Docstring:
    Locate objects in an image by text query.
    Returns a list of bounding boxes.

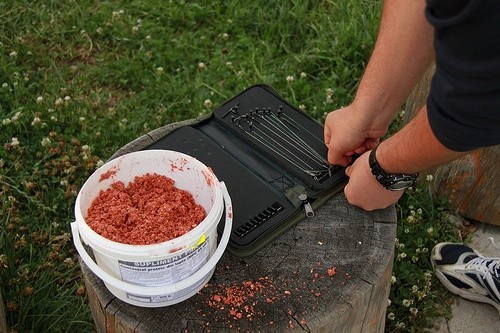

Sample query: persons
[324,0,500,313]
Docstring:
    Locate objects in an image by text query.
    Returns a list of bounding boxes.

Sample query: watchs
[369,144,419,191]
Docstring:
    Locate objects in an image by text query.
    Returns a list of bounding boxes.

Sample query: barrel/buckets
[69,150,233,308]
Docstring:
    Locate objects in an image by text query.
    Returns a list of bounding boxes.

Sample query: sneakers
[431,242,500,315]
